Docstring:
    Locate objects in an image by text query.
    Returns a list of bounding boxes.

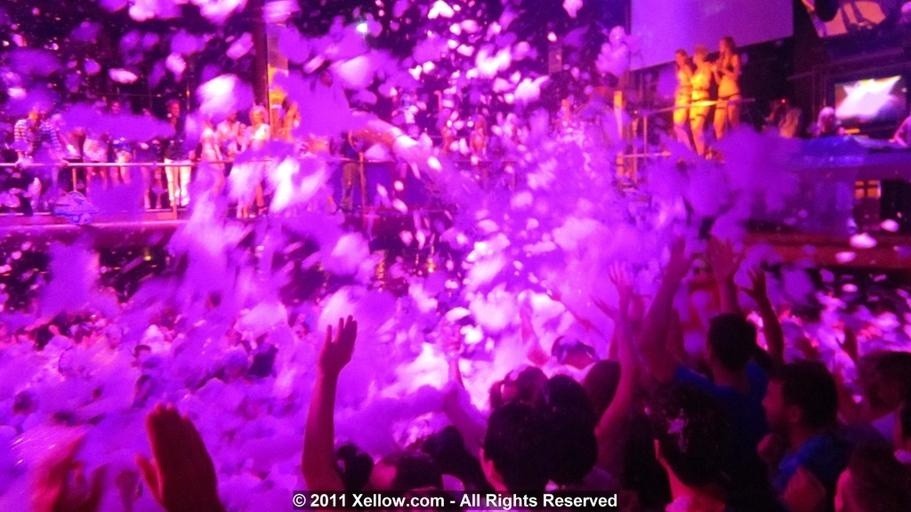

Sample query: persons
[0,35,909,512]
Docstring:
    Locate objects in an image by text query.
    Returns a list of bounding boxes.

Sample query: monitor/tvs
[833,73,901,125]
